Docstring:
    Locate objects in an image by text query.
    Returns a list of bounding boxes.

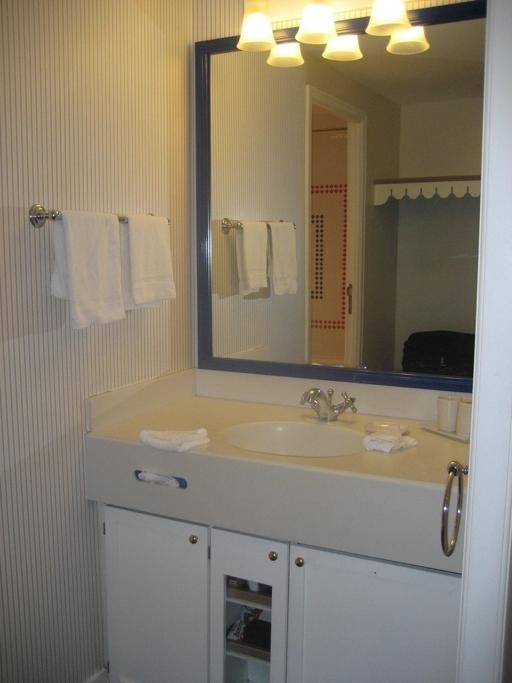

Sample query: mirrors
[194,15,486,392]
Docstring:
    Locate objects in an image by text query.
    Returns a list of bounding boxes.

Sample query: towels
[237,220,269,299]
[362,432,419,455]
[138,427,211,454]
[124,213,179,309]
[266,220,300,297]
[50,208,127,328]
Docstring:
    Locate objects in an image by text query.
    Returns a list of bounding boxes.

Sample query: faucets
[299,387,358,423]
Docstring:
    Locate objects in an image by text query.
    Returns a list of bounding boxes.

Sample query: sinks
[222,420,369,457]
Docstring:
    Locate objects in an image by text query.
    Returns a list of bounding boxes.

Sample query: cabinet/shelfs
[100,502,210,683]
[210,525,462,682]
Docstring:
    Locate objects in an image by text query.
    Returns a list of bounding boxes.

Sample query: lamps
[235,0,412,51]
[266,25,430,68]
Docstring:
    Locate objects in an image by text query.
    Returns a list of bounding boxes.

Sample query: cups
[435,395,460,432]
[456,400,472,438]
